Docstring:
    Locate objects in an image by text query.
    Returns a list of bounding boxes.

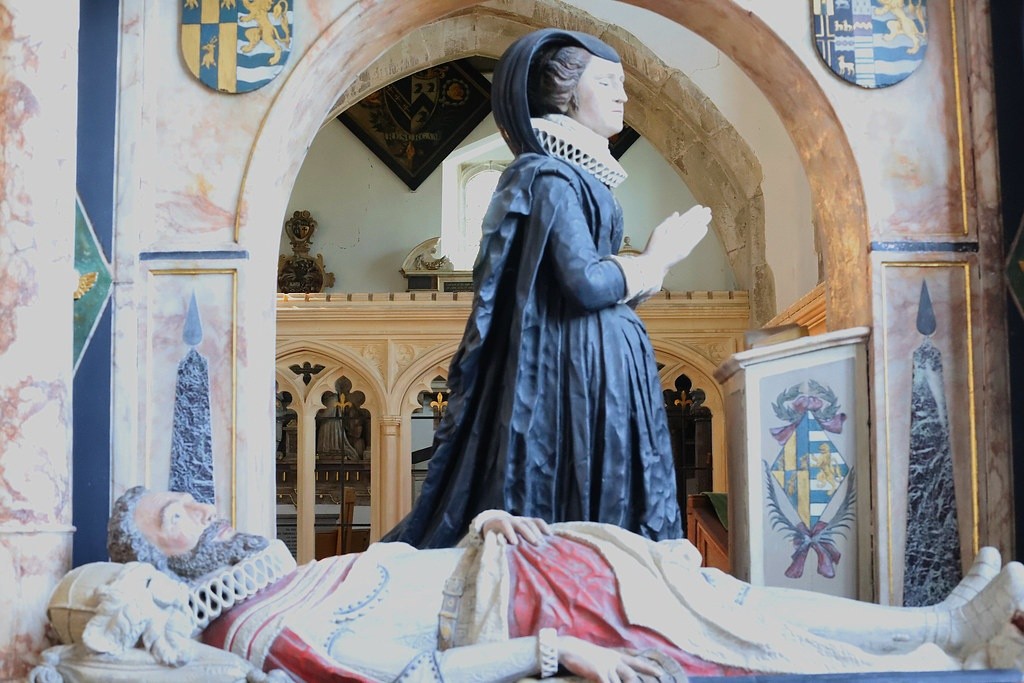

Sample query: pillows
[43,559,162,647]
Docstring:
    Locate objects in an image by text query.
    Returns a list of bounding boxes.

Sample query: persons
[106,485,1024,683]
[375,28,713,550]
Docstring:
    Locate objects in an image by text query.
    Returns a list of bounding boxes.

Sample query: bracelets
[538,629,559,681]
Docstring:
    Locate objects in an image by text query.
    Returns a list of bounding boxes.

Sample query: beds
[42,628,1024,683]
